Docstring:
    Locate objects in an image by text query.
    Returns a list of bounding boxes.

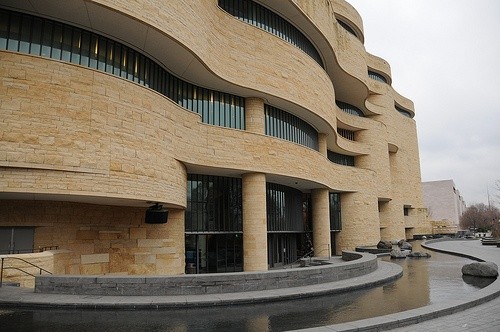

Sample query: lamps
[145,203,169,224]
